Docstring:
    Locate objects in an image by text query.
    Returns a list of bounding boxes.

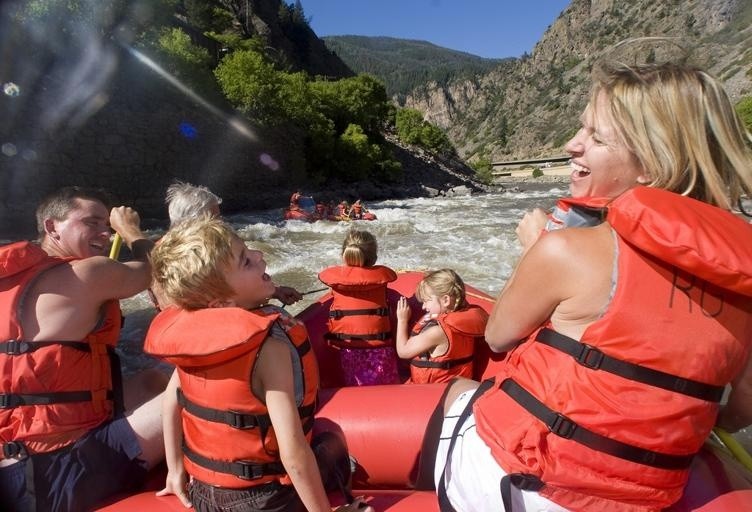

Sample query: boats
[89,272,750,512]
[284,202,379,222]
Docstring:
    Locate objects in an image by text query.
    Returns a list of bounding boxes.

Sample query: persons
[289,191,369,219]
[0,185,175,511]
[318,229,401,386]
[413,35,752,512]
[146,183,302,315]
[144,215,374,512]
[396,269,491,385]
